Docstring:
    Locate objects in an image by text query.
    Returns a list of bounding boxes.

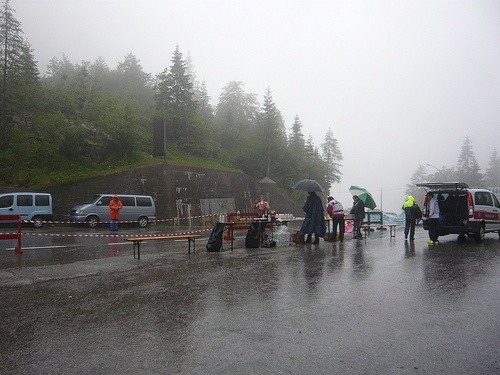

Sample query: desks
[216,219,275,252]
[282,218,360,246]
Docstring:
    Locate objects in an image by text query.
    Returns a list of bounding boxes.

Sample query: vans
[0,192,53,229]
[69,194,157,228]
[416,181,500,243]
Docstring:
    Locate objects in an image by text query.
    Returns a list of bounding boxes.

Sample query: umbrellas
[291,176,323,198]
[348,185,377,211]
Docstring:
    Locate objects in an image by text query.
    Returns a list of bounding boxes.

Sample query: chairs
[436,196,444,201]
[481,195,488,203]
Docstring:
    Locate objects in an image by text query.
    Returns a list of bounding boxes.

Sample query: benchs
[124,234,202,259]
[358,224,399,239]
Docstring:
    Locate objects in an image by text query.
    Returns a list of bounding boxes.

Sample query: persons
[299,192,327,245]
[349,194,365,238]
[109,193,126,231]
[326,196,346,243]
[425,191,441,246]
[402,195,423,241]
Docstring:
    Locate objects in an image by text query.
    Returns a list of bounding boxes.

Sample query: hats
[115,194,119,200]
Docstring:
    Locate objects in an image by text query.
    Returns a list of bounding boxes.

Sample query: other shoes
[111,228,118,231]
[331,236,336,241]
[410,236,415,241]
[339,236,344,242]
[404,232,408,239]
[304,240,311,243]
[352,234,362,239]
[426,238,440,245]
[312,240,319,244]
